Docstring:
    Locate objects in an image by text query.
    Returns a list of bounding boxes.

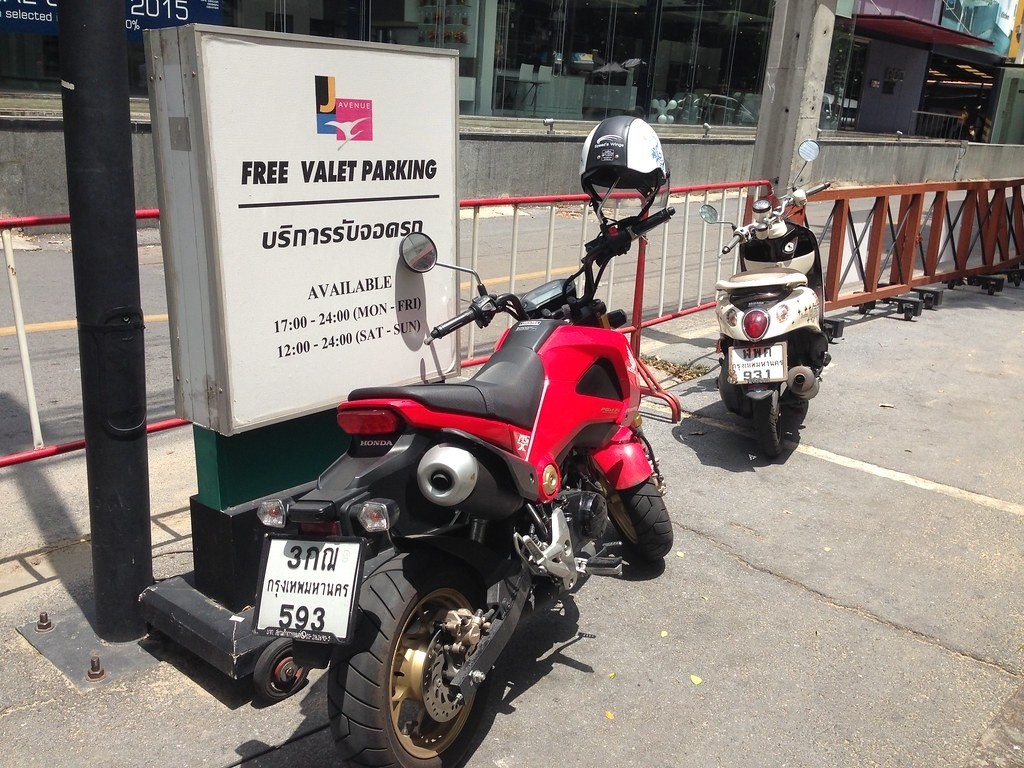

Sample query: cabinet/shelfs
[493,69,586,118]
[585,84,638,114]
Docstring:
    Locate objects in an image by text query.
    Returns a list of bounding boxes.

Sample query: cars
[689,93,754,126]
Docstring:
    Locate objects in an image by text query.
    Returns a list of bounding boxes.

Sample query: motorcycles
[246,176,675,768]
[698,139,831,460]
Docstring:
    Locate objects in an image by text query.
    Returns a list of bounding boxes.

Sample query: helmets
[579,115,667,189]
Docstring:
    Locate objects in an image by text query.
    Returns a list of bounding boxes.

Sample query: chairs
[506,63,553,118]
